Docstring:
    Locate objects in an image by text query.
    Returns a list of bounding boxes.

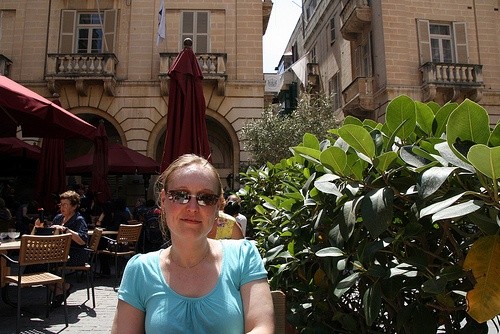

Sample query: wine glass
[0,232,8,241]
[8,232,20,241]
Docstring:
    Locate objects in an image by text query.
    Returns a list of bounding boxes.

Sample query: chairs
[0,216,142,334]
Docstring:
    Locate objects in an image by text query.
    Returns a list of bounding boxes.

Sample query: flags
[155,0,167,47]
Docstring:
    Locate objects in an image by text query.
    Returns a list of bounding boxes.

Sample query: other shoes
[51,281,73,308]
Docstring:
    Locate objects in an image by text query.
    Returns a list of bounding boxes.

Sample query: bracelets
[63,227,69,234]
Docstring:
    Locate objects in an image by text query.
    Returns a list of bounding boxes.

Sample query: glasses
[166,189,219,206]
[59,203,70,208]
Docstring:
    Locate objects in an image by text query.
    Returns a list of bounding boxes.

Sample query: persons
[110,153,277,334]
[0,183,247,307]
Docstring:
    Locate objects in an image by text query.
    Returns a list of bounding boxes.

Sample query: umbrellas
[0,74,160,212]
[160,37,214,175]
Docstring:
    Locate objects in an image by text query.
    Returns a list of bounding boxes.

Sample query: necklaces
[170,246,210,269]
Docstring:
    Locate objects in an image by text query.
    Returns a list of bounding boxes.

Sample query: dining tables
[87,230,119,281]
[0,239,60,301]
